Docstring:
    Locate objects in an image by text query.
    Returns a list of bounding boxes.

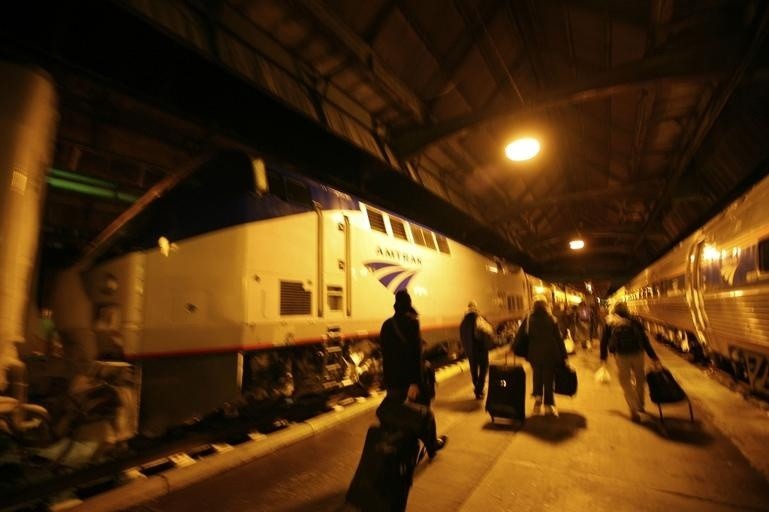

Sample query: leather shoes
[423,436,448,460]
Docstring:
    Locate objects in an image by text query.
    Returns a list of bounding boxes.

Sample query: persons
[459,298,487,401]
[598,300,662,423]
[551,298,611,357]
[380,289,450,462]
[526,300,560,418]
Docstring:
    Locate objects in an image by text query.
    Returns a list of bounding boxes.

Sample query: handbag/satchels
[374,397,434,439]
[513,313,532,357]
[645,361,688,406]
[553,364,578,396]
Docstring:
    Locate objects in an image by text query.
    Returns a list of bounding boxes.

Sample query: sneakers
[534,400,559,418]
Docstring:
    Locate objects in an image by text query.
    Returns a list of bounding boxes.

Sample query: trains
[90,161,590,420]
[601,176,768,396]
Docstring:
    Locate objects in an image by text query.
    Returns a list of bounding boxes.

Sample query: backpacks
[608,312,644,354]
[473,312,497,348]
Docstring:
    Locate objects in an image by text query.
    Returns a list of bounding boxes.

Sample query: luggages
[480,347,527,424]
[345,419,421,512]
